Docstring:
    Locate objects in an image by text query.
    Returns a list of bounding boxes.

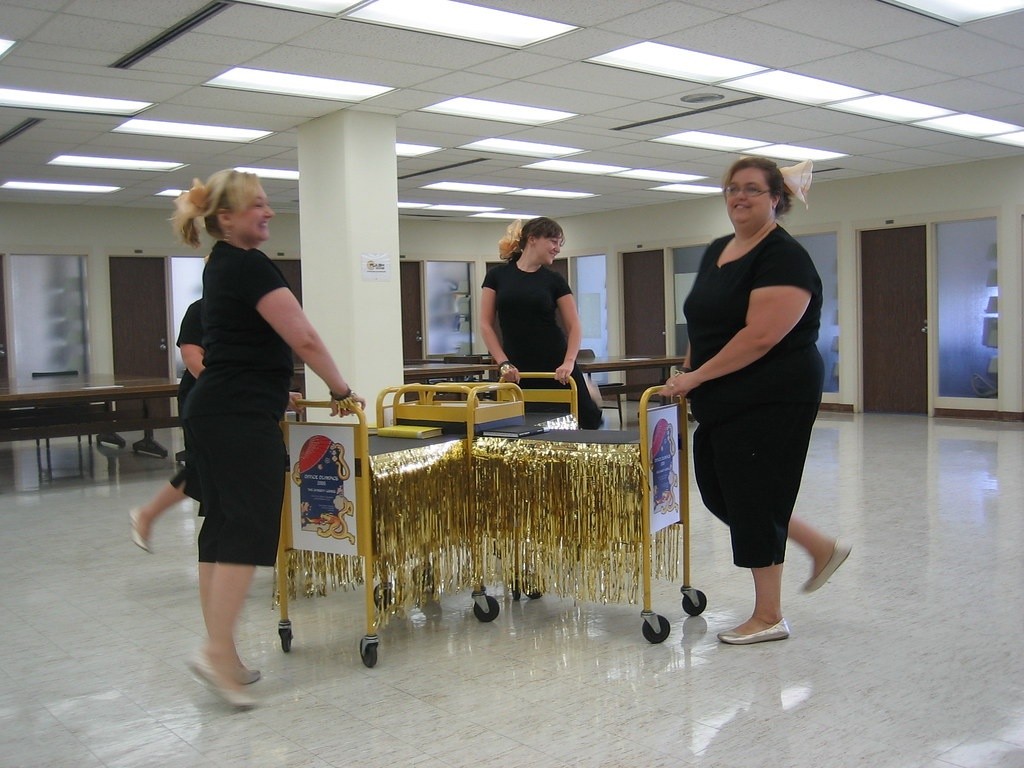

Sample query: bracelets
[329,384,360,410]
[498,360,515,374]
[674,367,691,376]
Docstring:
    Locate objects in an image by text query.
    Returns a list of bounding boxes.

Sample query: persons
[128,298,207,552]
[658,156,853,646]
[171,169,367,708]
[480,216,605,431]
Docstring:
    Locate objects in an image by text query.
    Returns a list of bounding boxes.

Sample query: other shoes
[798,534,853,595]
[183,646,269,707]
[128,506,152,554]
[716,617,790,644]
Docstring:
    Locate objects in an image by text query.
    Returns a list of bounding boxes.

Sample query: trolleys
[364,382,422,429]
[276,384,480,669]
[426,370,580,431]
[466,382,708,646]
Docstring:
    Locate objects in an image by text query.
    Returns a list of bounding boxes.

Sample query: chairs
[444,356,484,397]
[575,348,624,426]
[31,370,92,447]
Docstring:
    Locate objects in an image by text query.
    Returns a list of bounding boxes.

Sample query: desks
[285,345,701,432]
[0,372,186,466]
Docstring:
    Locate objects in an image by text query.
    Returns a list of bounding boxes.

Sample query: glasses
[724,185,771,197]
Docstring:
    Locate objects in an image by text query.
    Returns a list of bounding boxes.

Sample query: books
[377,424,443,439]
[482,425,550,438]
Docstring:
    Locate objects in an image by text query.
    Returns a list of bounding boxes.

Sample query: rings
[670,383,674,388]
